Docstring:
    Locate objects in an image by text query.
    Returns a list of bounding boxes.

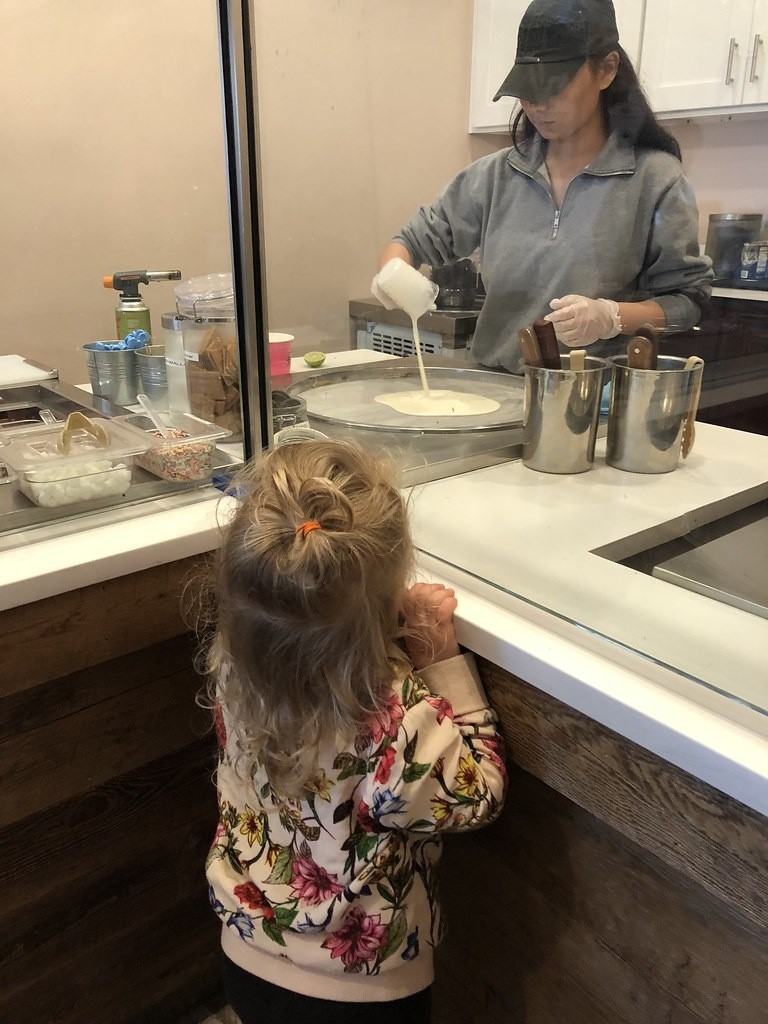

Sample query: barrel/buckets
[134,344,178,411]
[82,339,141,407]
[703,213,764,282]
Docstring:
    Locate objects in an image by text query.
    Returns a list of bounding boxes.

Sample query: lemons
[304,352,326,366]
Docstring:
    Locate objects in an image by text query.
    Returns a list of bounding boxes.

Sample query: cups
[375,256,441,319]
[519,352,612,474]
[606,354,702,474]
[268,332,295,376]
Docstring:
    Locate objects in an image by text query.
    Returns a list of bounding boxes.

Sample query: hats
[492,0,620,103]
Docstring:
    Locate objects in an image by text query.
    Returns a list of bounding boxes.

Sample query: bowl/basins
[436,287,477,311]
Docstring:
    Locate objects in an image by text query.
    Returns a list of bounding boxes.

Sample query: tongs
[680,356,704,459]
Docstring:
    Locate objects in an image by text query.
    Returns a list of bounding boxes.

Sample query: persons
[174,436,507,1023]
[371,0,714,375]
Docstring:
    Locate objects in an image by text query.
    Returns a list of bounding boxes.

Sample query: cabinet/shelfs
[705,298,768,437]
[659,296,726,424]
[468,0,767,136]
